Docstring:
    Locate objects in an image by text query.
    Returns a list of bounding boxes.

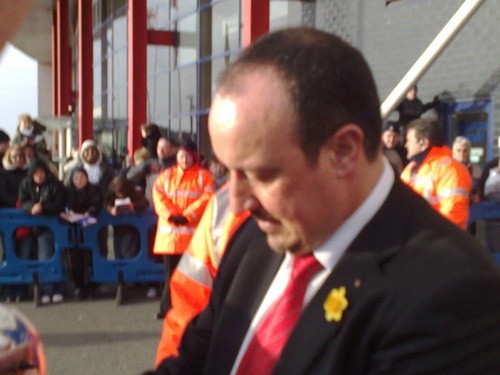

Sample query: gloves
[168,215,187,226]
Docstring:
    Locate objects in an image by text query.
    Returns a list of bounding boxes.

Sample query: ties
[235,254,323,375]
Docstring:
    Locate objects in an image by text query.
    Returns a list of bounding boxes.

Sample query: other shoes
[156,311,166,319]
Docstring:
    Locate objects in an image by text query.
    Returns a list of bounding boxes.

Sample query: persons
[140,24,500,375]
[0,113,229,320]
[382,84,500,232]
